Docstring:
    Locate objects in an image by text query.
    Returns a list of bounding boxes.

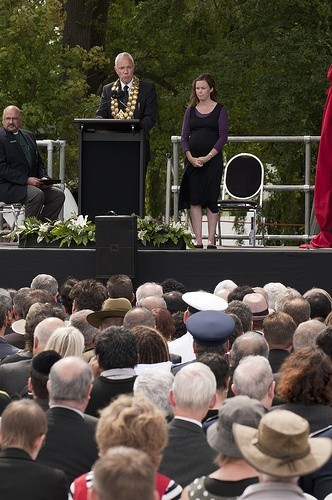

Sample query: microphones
[82,94,116,118]
[116,95,135,120]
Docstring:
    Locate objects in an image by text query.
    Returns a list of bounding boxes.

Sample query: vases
[19,234,185,249]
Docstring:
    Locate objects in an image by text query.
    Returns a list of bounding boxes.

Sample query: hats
[232,409,332,477]
[251,287,269,307]
[207,395,272,457]
[32,349,63,375]
[264,282,286,309]
[213,280,238,303]
[86,298,133,329]
[243,293,275,321]
[185,310,235,346]
[12,302,51,335]
[182,292,229,313]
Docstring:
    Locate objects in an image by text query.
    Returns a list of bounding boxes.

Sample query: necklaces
[110,75,140,120]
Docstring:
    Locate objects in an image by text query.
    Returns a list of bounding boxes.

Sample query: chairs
[217,152,267,248]
[0,203,26,246]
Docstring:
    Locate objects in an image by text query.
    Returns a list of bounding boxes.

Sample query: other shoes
[207,244,217,249]
[194,244,203,248]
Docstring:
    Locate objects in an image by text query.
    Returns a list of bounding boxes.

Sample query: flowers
[3,215,197,250]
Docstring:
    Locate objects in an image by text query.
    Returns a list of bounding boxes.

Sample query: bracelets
[209,151,213,157]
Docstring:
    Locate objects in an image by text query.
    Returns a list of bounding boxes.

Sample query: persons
[95,51,158,178]
[0,273,332,500]
[181,72,228,249]
[0,104,65,226]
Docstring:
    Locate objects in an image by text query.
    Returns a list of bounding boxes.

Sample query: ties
[123,86,129,113]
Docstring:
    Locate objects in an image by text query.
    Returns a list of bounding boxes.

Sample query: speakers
[94,214,137,279]
